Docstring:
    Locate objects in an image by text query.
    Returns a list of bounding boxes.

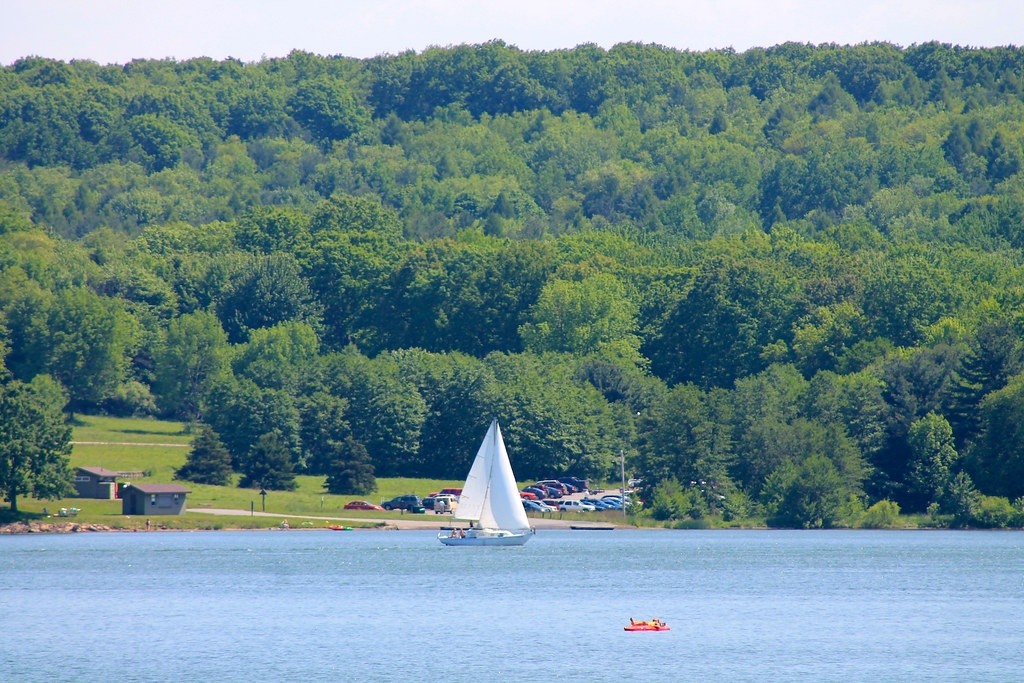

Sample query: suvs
[381,495,422,512]
[423,493,457,510]
[430,488,463,500]
[434,495,459,514]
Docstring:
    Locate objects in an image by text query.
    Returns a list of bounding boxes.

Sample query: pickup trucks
[558,476,589,492]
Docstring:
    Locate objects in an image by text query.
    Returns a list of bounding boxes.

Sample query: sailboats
[437,410,536,547]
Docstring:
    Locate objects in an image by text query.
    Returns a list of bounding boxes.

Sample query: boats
[624,618,670,631]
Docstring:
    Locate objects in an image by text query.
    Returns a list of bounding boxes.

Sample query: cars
[519,490,536,500]
[522,486,547,500]
[563,482,578,495]
[343,500,376,509]
[522,478,647,514]
